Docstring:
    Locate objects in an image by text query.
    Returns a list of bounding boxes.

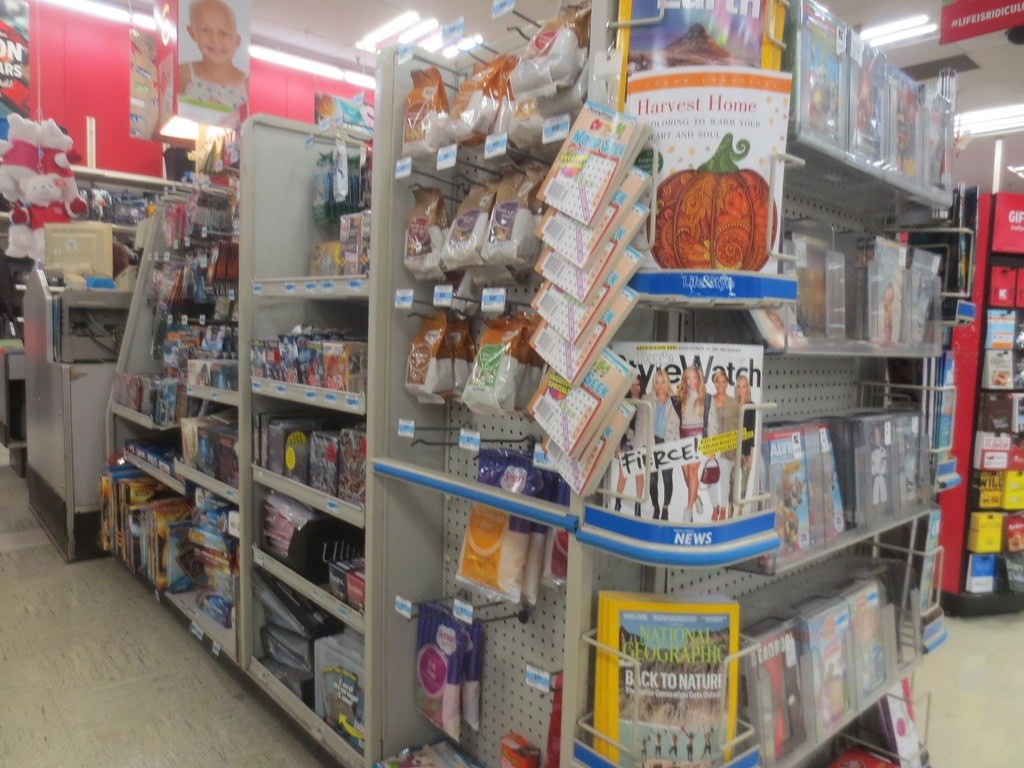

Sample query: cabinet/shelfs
[0,1,1023,768]
